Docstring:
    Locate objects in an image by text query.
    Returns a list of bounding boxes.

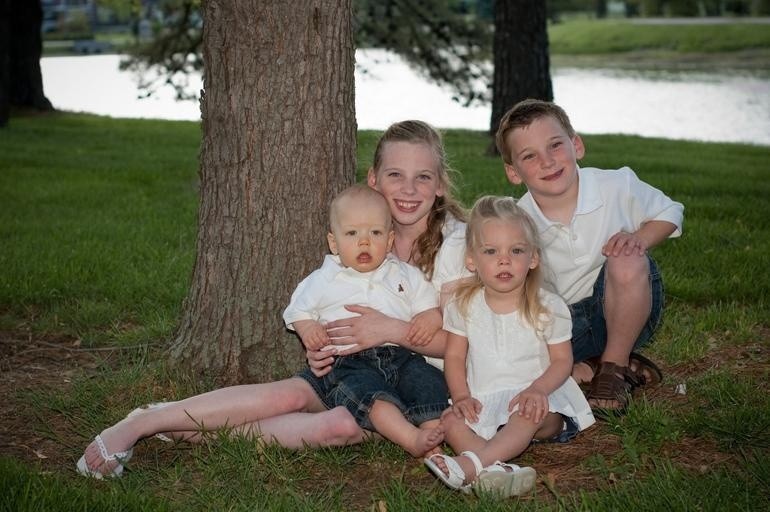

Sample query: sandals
[473,459,536,500]
[424,451,484,493]
[586,352,662,418]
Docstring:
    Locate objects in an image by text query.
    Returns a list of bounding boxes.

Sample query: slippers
[77,402,174,481]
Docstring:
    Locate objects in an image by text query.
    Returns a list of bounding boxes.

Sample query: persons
[494,95,684,419]
[279,180,454,460]
[77,119,484,484]
[424,193,600,506]
